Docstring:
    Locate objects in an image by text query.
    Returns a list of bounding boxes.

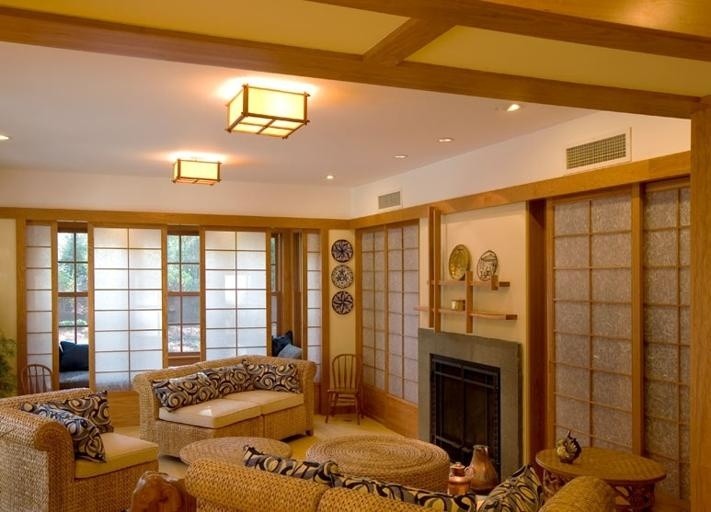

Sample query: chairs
[324,352,364,425]
[21,362,53,393]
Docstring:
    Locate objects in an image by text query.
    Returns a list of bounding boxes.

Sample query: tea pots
[449,462,477,496]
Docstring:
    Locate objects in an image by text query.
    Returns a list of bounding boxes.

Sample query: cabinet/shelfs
[412,278,519,334]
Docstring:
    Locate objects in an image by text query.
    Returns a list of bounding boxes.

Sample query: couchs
[182,456,617,512]
[0,386,160,512]
[131,354,317,463]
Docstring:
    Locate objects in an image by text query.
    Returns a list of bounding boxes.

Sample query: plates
[477,250,498,282]
[448,244,470,280]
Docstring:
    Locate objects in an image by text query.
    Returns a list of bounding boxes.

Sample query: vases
[466,444,499,497]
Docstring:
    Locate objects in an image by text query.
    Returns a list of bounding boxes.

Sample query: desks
[536,446,667,510]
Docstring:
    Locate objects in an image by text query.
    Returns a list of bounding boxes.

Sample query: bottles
[469,444,500,493]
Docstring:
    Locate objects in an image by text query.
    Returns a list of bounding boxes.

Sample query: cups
[452,300,465,311]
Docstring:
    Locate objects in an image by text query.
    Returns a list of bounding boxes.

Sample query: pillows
[22,400,107,467]
[151,371,224,413]
[242,442,340,494]
[330,469,477,511]
[478,463,547,512]
[37,391,115,434]
[59,339,89,371]
[201,362,254,394]
[270,331,303,359]
[242,357,301,396]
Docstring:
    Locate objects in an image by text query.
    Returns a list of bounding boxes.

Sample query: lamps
[223,83,312,139]
[171,157,222,188]
